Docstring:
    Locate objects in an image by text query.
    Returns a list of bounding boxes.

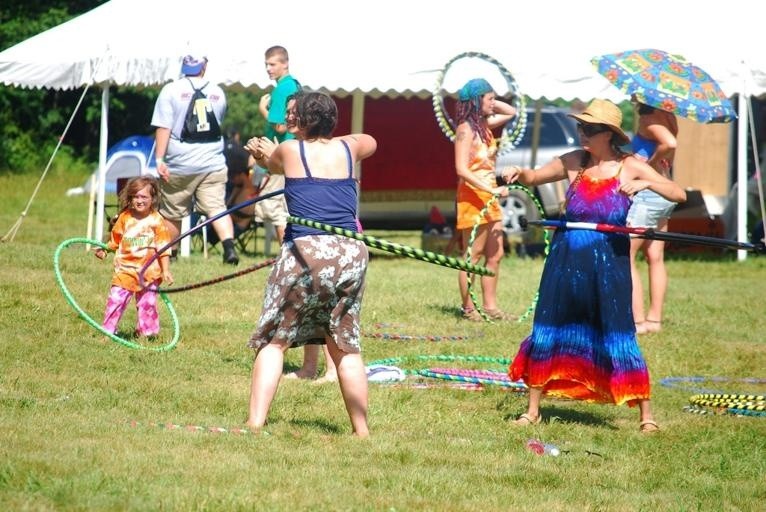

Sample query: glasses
[576,123,606,137]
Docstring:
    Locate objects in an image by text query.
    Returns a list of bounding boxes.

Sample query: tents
[83,134,160,192]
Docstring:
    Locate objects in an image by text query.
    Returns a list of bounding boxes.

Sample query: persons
[150,51,240,267]
[243,91,378,438]
[244,93,341,384]
[625,91,680,336]
[451,77,518,320]
[501,96,688,437]
[253,45,304,245]
[91,175,175,345]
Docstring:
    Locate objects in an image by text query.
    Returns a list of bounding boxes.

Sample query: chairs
[191,184,258,257]
[96,176,129,228]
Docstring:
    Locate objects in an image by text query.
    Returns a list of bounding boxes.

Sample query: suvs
[493,105,587,248]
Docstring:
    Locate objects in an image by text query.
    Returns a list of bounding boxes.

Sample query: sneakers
[223,248,238,264]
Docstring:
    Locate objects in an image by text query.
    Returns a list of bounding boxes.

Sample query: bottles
[527,438,562,456]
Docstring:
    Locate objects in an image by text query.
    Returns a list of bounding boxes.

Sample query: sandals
[512,412,542,426]
[482,305,517,321]
[640,420,658,432]
[461,307,484,322]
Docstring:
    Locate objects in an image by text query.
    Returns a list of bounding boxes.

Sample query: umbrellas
[590,49,739,127]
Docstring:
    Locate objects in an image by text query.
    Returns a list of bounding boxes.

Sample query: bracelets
[254,154,265,161]
[154,157,166,165]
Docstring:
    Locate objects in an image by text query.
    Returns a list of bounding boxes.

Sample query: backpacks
[179,78,221,143]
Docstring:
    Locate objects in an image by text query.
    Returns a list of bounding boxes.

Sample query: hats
[182,55,206,76]
[567,99,632,146]
